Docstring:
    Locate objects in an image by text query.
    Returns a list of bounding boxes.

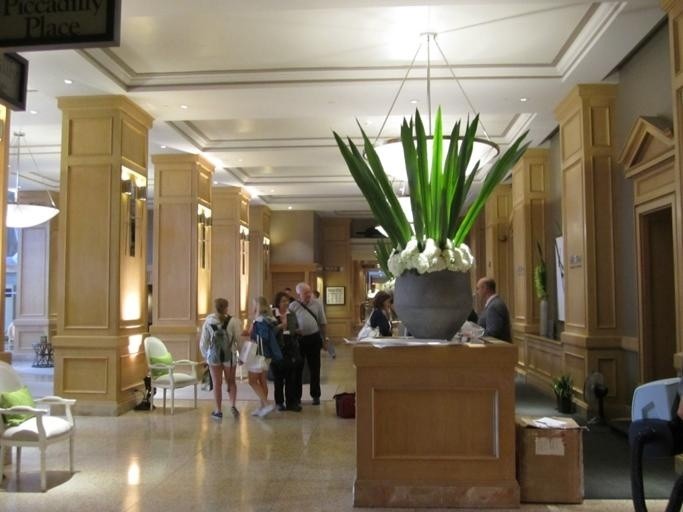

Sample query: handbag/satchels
[271,341,304,375]
[334,392,354,418]
[239,341,257,364]
[248,355,265,373]
[357,323,381,337]
[201,368,213,391]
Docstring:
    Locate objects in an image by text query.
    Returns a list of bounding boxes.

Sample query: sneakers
[212,411,222,418]
[251,408,262,416]
[287,405,301,411]
[277,404,284,411]
[312,398,319,405]
[230,407,239,416]
[260,406,274,417]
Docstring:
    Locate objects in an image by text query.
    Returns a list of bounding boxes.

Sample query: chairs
[0,361,77,491]
[142,334,198,413]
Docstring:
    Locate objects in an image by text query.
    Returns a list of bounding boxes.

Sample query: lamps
[121,177,147,202]
[6,124,57,229]
[365,30,496,179]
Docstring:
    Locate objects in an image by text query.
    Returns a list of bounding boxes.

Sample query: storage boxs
[517,414,587,505]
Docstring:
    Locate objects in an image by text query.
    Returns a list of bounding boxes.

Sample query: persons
[239,295,285,418]
[467,310,478,323]
[199,297,242,420]
[367,291,393,336]
[270,282,335,412]
[476,277,512,342]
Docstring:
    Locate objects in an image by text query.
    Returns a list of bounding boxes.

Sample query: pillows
[150,352,174,376]
[0,386,36,425]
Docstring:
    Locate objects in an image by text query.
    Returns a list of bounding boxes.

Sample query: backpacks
[207,315,234,363]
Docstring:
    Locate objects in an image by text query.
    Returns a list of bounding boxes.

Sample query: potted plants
[330,107,533,340]
[550,373,573,415]
[533,241,549,337]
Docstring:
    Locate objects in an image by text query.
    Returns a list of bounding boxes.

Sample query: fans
[581,371,608,428]
